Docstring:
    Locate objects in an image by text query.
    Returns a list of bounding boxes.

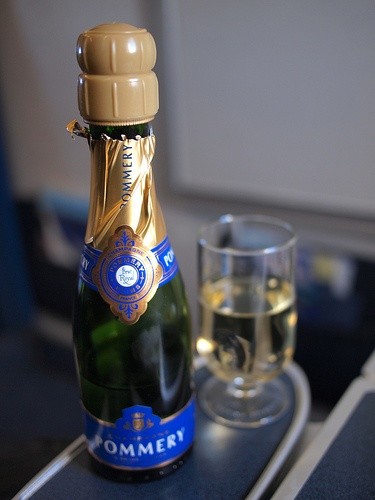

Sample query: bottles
[70,23,196,484]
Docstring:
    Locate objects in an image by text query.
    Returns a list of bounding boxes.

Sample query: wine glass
[195,214,297,429]
